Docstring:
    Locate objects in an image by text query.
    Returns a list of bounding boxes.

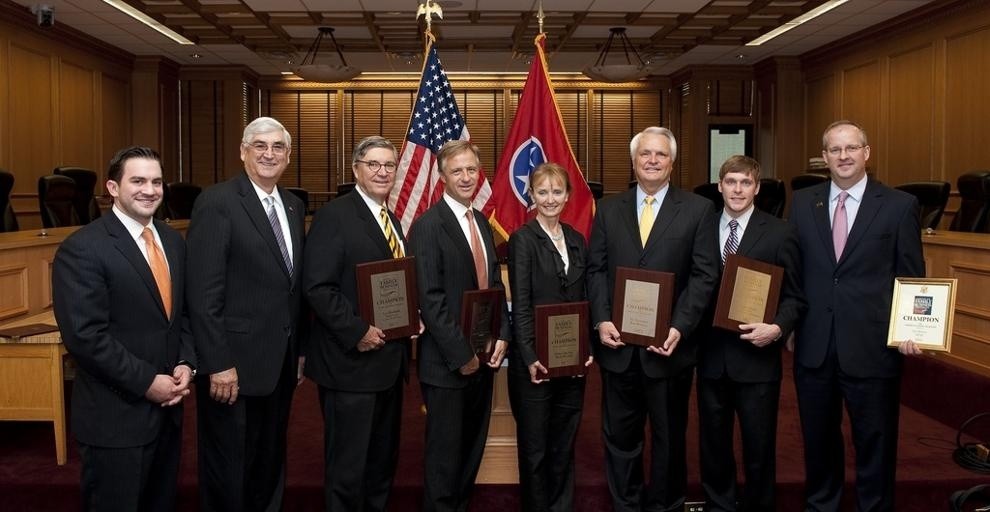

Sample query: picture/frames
[886,277,958,353]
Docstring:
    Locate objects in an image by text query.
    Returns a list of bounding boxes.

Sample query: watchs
[177,359,197,379]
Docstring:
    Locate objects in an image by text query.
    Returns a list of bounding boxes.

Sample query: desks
[0,310,69,466]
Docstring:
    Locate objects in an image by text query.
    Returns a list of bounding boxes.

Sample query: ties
[380,207,404,258]
[832,192,848,261]
[265,196,293,277]
[723,220,739,263]
[142,226,172,321]
[640,196,655,247]
[466,210,487,289]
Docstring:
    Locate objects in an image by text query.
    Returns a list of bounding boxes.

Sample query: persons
[298,135,425,511]
[504,162,594,512]
[585,124,719,511]
[406,141,512,512]
[50,146,198,511]
[693,154,805,512]
[186,115,306,512]
[788,120,937,511]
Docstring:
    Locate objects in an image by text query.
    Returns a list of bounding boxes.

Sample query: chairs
[896,181,951,230]
[588,182,603,200]
[38,174,80,229]
[55,168,102,221]
[285,188,310,208]
[949,172,990,232]
[2,172,21,234]
[166,182,203,220]
[694,183,725,215]
[754,176,786,219]
[335,183,355,197]
[791,174,829,190]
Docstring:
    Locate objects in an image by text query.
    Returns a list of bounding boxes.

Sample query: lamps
[583,27,657,83]
[290,27,361,82]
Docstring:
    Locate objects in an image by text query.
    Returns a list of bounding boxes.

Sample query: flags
[490,31,598,255]
[383,34,494,242]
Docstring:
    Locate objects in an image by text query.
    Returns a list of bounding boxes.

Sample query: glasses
[356,159,398,174]
[249,141,286,155]
[829,144,864,153]
